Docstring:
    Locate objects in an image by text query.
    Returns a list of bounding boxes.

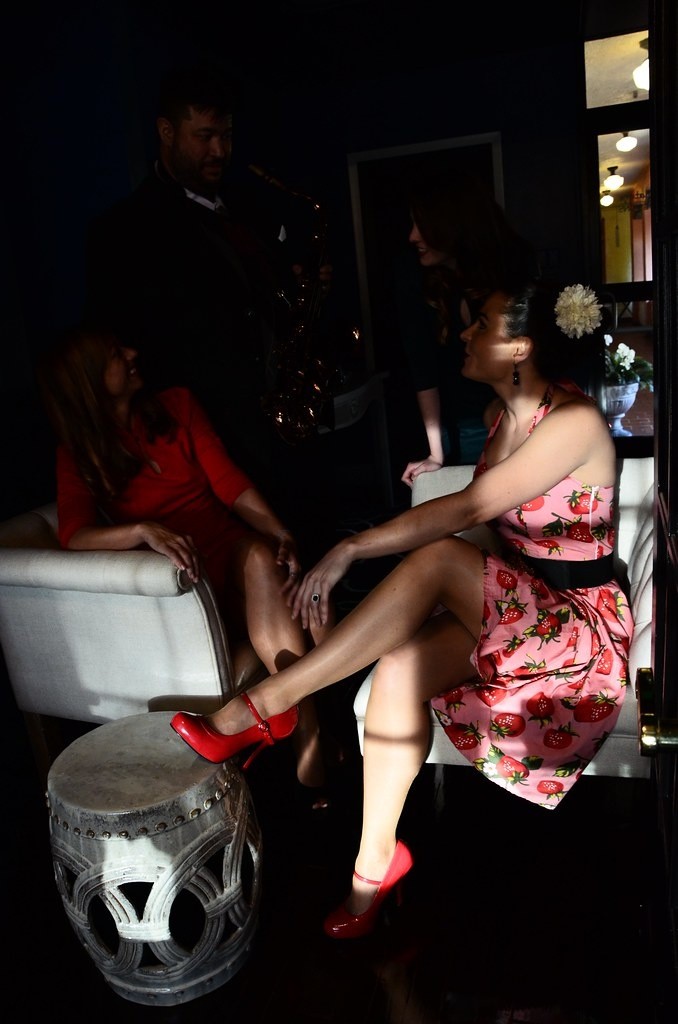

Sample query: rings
[289,573,298,579]
[310,593,319,603]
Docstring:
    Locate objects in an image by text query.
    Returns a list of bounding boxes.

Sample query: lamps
[616,133,638,152]
[604,166,624,190]
[633,38,649,91]
[600,190,614,207]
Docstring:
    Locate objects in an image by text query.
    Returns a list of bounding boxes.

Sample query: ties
[214,206,231,221]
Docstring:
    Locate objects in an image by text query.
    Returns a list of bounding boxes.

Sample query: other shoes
[295,758,333,811]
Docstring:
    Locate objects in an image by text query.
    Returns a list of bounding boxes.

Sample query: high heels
[323,838,416,941]
[169,691,301,770]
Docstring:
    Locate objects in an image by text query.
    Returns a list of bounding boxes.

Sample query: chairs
[353,456,656,819]
[0,501,262,811]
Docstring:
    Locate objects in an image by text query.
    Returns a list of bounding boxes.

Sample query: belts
[517,550,616,593]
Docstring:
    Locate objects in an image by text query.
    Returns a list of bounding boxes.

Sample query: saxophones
[247,161,373,445]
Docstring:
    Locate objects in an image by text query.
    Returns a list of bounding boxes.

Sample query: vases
[585,383,639,437]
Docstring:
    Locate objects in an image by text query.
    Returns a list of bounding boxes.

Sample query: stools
[43,712,271,1008]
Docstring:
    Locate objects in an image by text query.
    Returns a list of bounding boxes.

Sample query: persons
[169,263,635,938]
[35,326,351,822]
[401,185,538,488]
[85,97,265,490]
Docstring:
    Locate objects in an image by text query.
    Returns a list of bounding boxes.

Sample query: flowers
[601,334,653,393]
[554,284,603,339]
